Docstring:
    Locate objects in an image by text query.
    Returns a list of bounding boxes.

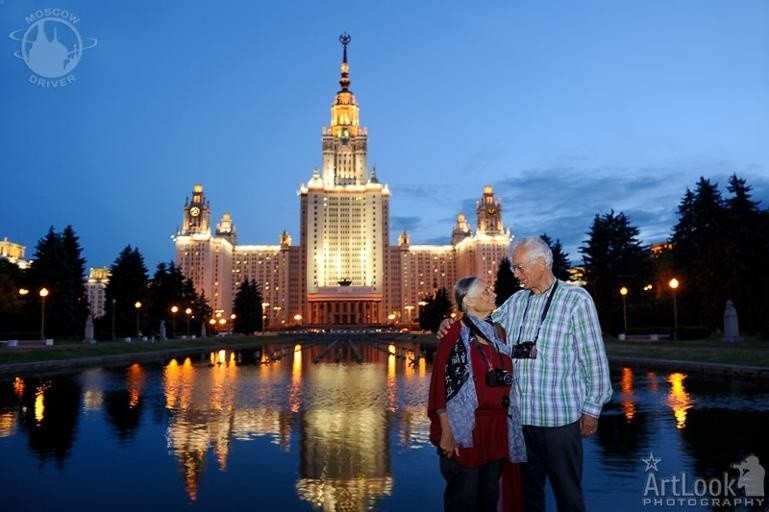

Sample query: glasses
[510,261,531,273]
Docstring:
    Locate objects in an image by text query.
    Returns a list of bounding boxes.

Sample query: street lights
[184,307,192,336]
[38,287,50,345]
[386,300,429,333]
[618,285,628,328]
[170,305,179,339]
[111,298,117,342]
[207,301,302,335]
[668,277,680,340]
[133,301,143,338]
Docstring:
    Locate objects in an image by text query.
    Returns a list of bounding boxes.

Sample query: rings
[590,429,595,434]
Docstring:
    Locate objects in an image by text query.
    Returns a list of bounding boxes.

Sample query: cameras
[511,344,537,359]
[487,368,513,386]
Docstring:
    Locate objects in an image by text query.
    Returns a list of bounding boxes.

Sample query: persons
[423,274,517,512]
[722,300,740,337]
[433,236,614,512]
